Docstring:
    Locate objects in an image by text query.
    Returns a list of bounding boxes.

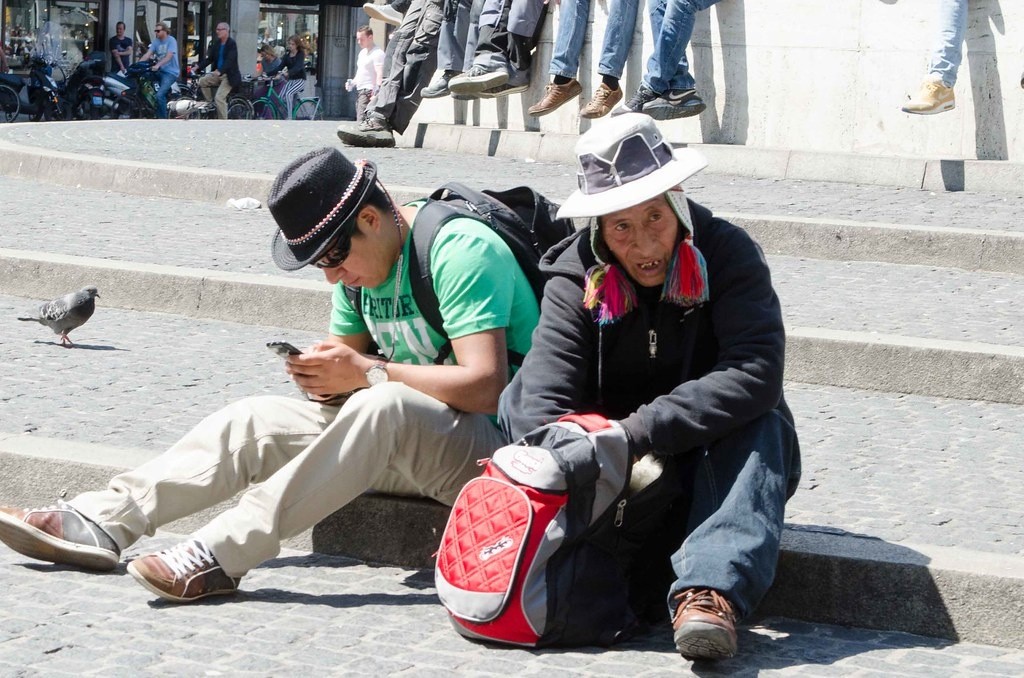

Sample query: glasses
[216,28,226,32]
[309,214,356,270]
[154,29,164,33]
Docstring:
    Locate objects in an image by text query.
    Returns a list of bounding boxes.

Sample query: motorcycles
[0,22,136,122]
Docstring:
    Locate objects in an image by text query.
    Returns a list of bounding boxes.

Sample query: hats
[266,146,377,272]
[554,113,708,219]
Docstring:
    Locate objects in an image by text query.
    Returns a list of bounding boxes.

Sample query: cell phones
[266,342,303,360]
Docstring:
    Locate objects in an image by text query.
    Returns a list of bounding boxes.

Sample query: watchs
[366,360,388,387]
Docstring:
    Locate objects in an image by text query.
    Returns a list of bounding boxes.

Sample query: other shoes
[337,113,396,148]
[528,77,582,117]
[580,82,623,119]
[363,2,404,25]
[199,102,216,111]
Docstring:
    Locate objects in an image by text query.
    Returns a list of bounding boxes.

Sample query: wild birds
[17,285,101,349]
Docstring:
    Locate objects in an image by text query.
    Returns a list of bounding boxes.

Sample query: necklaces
[387,205,405,363]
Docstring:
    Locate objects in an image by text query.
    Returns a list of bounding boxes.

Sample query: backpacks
[430,413,667,648]
[408,182,577,367]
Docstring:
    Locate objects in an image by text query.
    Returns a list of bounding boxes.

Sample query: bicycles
[111,56,254,120]
[0,83,20,123]
[243,70,323,120]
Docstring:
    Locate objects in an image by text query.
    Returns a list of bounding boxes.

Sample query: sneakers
[451,92,478,101]
[609,83,660,119]
[1,489,121,569]
[127,539,240,601]
[899,73,955,114]
[420,70,461,98]
[448,63,508,93]
[669,583,736,661]
[642,88,706,120]
[473,84,531,99]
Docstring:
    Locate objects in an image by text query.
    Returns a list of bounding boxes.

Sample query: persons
[109,21,133,119]
[192,22,241,119]
[345,26,385,124]
[612,0,719,121]
[261,36,307,120]
[498,113,802,659]
[0,40,9,73]
[529,0,638,118]
[880,0,969,116]
[139,23,180,119]
[0,148,539,602]
[337,0,549,146]
